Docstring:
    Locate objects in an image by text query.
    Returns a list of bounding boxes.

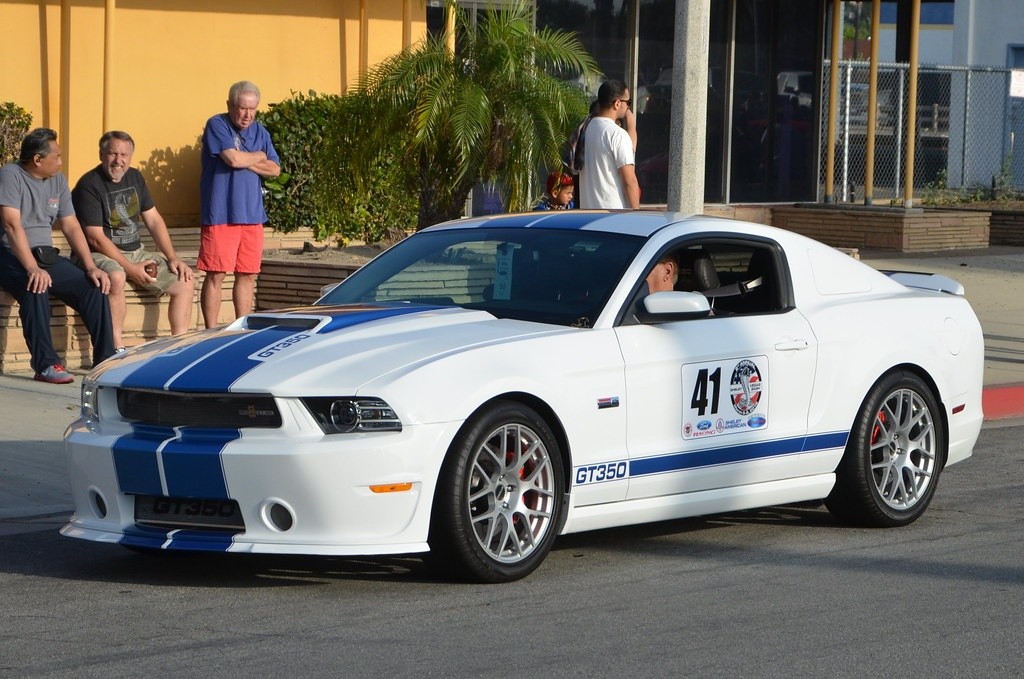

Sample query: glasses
[611,99,632,107]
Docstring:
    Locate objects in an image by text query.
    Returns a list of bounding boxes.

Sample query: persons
[70,132,193,353]
[568,80,639,209]
[196,82,281,328]
[0,128,116,384]
[533,171,574,211]
[644,256,678,294]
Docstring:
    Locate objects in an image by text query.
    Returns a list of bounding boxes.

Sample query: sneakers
[114,347,126,353]
[33,365,74,384]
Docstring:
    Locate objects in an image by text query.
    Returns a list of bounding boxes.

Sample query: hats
[544,172,574,195]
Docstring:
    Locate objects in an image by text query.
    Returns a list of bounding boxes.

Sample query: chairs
[539,249,723,312]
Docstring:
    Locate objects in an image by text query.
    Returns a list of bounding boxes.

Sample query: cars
[58,208,987,584]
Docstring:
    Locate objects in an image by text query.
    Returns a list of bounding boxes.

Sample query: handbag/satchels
[31,246,60,268]
